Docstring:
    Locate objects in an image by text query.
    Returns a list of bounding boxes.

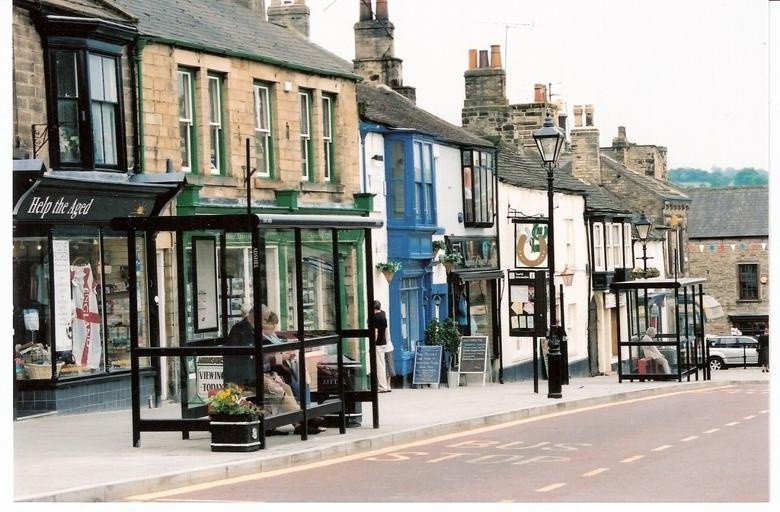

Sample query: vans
[698,334,759,370]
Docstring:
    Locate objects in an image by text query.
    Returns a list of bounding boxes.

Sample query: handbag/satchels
[291,360,311,385]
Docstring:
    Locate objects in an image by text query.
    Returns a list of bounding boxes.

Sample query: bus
[635,292,732,368]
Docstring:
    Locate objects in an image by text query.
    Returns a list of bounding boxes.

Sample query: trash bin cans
[316,353,362,428]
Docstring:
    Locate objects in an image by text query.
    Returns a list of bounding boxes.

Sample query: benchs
[638,356,660,375]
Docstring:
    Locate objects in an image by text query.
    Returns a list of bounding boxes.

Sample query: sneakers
[294,417,325,434]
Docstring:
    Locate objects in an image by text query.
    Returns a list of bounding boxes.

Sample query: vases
[211,414,260,452]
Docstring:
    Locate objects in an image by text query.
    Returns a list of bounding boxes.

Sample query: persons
[755,326,769,372]
[369,300,393,393]
[260,310,326,427]
[221,303,323,436]
[379,309,397,392]
[642,326,673,374]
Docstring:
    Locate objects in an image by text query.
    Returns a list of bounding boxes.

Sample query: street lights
[634,210,652,330]
[533,107,567,399]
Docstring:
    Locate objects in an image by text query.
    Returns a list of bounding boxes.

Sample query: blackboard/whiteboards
[412,346,443,384]
[457,336,488,373]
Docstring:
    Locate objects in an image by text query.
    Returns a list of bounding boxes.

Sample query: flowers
[209,383,260,413]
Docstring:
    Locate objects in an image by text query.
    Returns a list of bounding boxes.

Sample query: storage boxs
[24,361,65,380]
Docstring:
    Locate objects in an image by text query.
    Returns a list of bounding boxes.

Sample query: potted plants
[378,260,400,284]
[424,319,461,389]
[431,240,463,275]
